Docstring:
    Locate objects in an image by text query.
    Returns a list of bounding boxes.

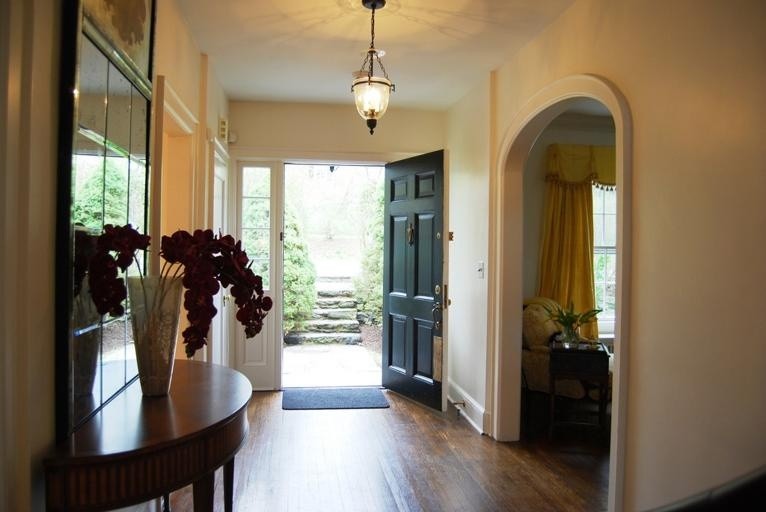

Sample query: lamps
[350,0,396,135]
[330,165,334,175]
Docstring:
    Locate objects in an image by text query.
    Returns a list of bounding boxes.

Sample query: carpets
[282,387,393,408]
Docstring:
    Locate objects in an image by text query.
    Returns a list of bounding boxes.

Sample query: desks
[44,359,253,511]
[548,342,609,429]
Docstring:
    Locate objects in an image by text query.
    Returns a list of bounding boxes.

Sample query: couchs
[522,297,614,401]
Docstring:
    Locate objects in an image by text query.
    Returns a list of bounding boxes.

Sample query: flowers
[85,223,273,358]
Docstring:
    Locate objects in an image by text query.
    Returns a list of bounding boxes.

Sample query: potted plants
[542,300,603,350]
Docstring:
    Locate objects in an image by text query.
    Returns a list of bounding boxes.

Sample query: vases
[126,276,185,397]
